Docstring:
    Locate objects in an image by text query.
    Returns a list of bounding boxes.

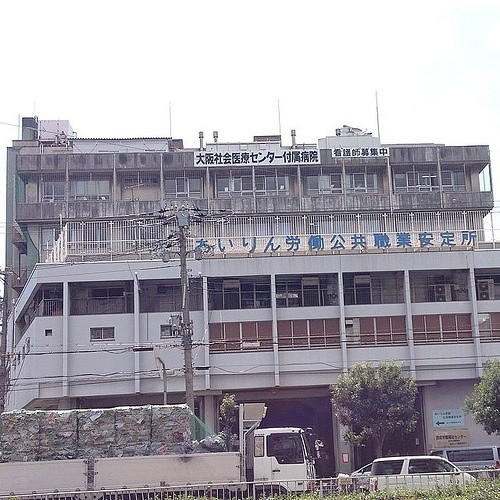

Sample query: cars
[341,464,370,495]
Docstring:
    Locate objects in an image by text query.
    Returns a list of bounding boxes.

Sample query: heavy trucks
[0,424,323,498]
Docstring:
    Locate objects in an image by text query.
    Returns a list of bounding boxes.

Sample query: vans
[429,446,500,479]
[371,456,476,495]
